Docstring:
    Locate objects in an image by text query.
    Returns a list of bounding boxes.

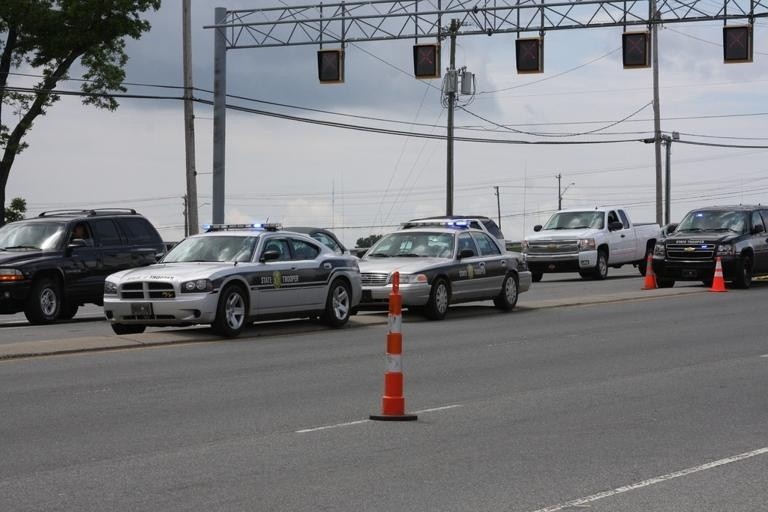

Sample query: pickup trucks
[522,206,662,281]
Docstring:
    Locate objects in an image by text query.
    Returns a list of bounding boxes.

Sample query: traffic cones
[707,255,729,294]
[640,255,658,291]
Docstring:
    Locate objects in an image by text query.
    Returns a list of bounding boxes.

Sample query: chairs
[408,237,481,257]
[565,216,614,228]
[215,242,306,262]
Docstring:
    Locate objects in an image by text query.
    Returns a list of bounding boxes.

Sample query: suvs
[651,204,768,287]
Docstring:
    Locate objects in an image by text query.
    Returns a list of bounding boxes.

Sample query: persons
[70,224,86,245]
